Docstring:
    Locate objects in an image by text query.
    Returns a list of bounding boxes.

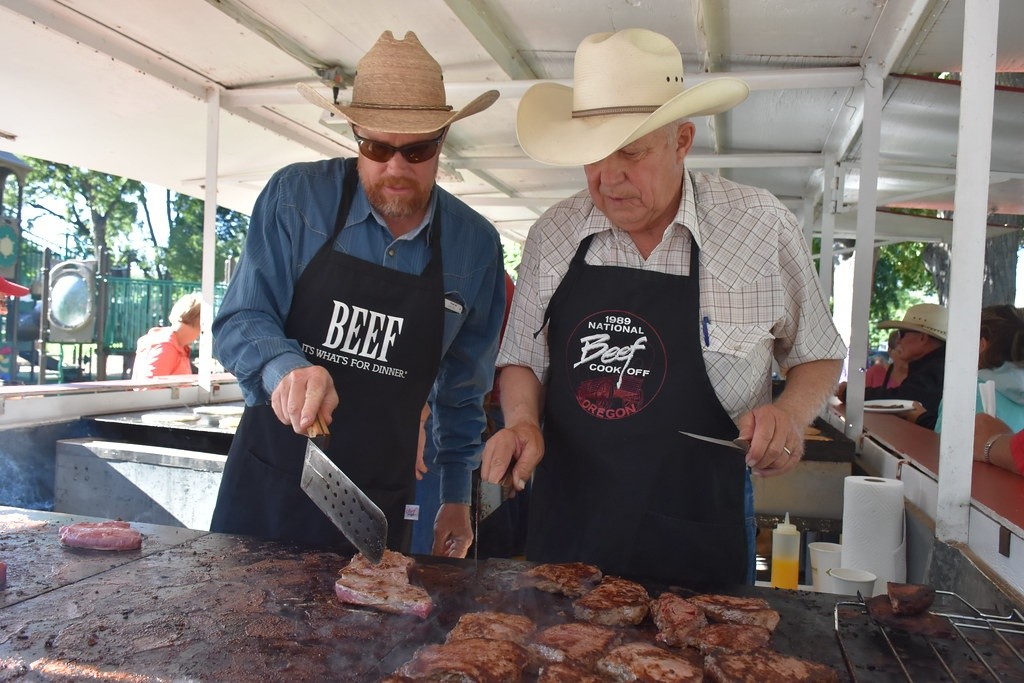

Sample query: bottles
[771,512,800,591]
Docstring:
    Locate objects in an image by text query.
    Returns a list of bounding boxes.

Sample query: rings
[783,446,791,455]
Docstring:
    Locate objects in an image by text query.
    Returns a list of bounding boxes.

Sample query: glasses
[898,330,917,339]
[352,123,446,163]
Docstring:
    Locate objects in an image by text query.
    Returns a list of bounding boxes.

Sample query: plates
[863,399,922,414]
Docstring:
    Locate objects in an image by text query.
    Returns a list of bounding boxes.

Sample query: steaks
[867,581,956,636]
[335,548,433,619]
[380,563,852,683]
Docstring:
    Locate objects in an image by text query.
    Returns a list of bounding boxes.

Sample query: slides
[19,342,62,371]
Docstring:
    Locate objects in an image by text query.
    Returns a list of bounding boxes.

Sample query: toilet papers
[841,475,907,597]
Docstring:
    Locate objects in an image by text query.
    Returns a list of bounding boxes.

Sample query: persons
[132,294,202,392]
[211,28,507,556]
[410,268,515,557]
[837,303,1024,480]
[481,30,847,582]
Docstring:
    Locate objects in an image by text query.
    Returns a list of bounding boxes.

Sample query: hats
[296,31,500,133]
[515,28,751,167]
[0,276,30,297]
[877,304,947,341]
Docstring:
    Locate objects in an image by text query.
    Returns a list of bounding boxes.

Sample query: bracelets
[984,433,1008,464]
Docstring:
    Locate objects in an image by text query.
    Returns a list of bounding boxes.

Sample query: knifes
[678,430,751,455]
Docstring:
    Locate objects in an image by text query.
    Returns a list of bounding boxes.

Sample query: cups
[829,567,877,597]
[808,542,842,594]
